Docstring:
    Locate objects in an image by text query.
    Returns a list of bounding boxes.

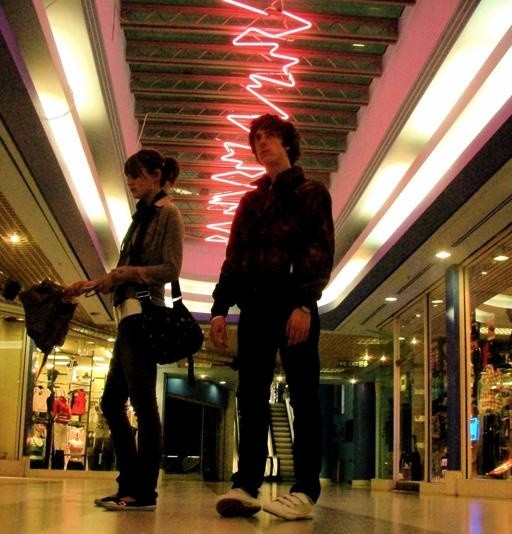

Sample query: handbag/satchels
[139,296,204,365]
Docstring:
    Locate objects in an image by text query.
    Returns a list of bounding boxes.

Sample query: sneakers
[264,492,315,519]
[215,489,261,516]
[96,492,157,511]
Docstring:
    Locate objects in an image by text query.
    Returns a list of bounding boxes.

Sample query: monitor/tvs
[469,417,485,441]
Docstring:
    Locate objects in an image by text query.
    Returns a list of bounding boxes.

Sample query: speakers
[3,277,20,301]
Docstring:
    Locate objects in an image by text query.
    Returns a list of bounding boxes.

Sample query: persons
[64,146,185,512]
[206,111,337,521]
